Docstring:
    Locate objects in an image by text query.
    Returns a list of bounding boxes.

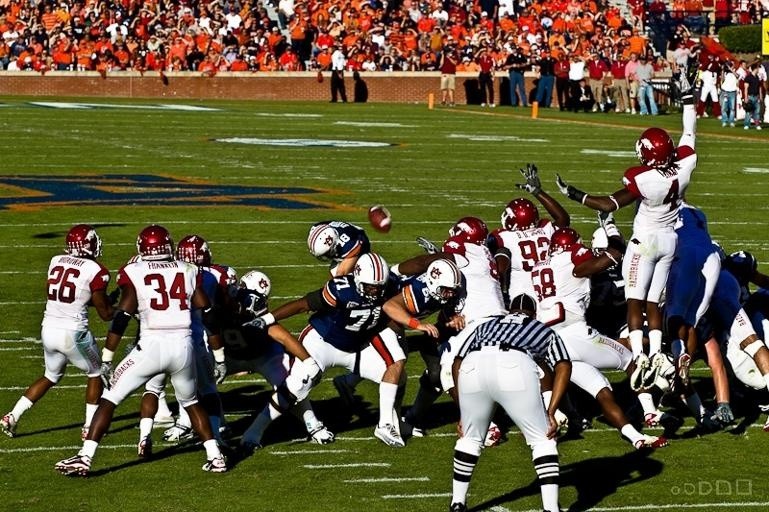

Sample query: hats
[511,294,537,311]
[482,12,488,17]
[74,18,80,22]
[273,27,279,31]
[522,26,529,31]
[448,35,454,40]
[322,45,328,50]
[465,37,472,41]
[535,35,542,39]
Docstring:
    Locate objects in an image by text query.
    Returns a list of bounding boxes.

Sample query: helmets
[550,228,582,252]
[591,227,609,248]
[449,217,489,246]
[354,253,389,299]
[240,270,271,298]
[502,198,539,231]
[426,259,462,304]
[136,225,211,267]
[636,128,675,168]
[66,224,104,258]
[306,225,339,258]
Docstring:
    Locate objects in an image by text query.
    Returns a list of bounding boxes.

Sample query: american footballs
[368,202,392,234]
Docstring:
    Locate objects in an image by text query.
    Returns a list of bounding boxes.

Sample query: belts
[469,344,527,354]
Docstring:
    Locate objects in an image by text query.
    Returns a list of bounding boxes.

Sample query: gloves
[555,172,587,204]
[105,288,120,306]
[598,210,620,238]
[100,347,114,390]
[417,237,439,254]
[241,313,275,332]
[303,357,322,387]
[515,164,541,194]
[212,346,227,386]
[674,68,699,104]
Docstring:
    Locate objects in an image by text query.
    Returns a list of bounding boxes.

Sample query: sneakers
[633,404,733,451]
[697,111,709,119]
[607,96,611,104]
[334,375,358,410]
[201,458,227,473]
[81,426,91,443]
[135,416,262,454]
[481,103,495,108]
[449,498,468,511]
[440,102,455,107]
[0,413,17,438]
[484,421,501,447]
[763,415,769,432]
[374,414,425,447]
[55,456,91,476]
[592,104,637,114]
[716,112,763,130]
[553,409,588,440]
[630,352,691,394]
[307,422,333,446]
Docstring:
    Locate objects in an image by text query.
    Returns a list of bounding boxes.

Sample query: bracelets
[99,345,113,359]
[567,185,589,205]
[388,262,402,279]
[257,310,278,328]
[405,316,421,331]
[682,89,696,106]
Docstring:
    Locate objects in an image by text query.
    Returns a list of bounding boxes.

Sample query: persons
[1,72,769,477]
[0,0,769,133]
[445,294,571,510]
